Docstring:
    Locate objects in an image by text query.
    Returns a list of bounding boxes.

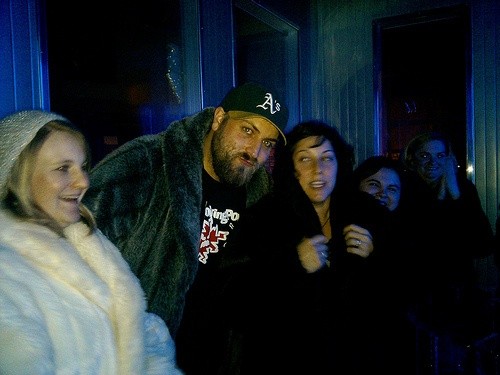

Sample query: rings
[321,251,327,258]
[356,240,362,247]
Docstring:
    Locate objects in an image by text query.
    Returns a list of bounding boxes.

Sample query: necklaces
[321,218,329,229]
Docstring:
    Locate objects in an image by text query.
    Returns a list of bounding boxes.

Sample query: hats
[0,109,70,195]
[219,81,290,147]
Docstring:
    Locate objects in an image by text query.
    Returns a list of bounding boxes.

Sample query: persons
[174,120,419,375]
[405,136,495,375]
[81,82,289,340]
[0,111,182,375]
